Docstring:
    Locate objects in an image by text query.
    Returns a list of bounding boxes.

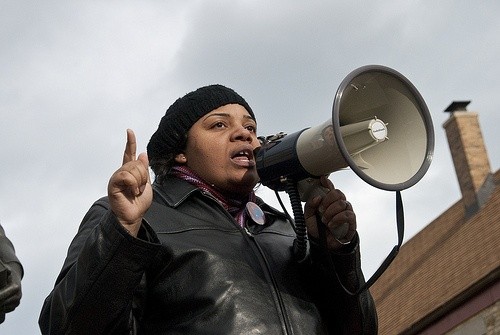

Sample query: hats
[147,84,258,164]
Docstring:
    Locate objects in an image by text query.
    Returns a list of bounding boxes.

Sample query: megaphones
[252,65,435,241]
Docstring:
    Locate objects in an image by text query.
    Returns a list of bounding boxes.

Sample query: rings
[344,201,351,210]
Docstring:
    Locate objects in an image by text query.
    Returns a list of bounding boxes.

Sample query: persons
[38,84,379,335]
[322,125,347,171]
[0,224,25,324]
